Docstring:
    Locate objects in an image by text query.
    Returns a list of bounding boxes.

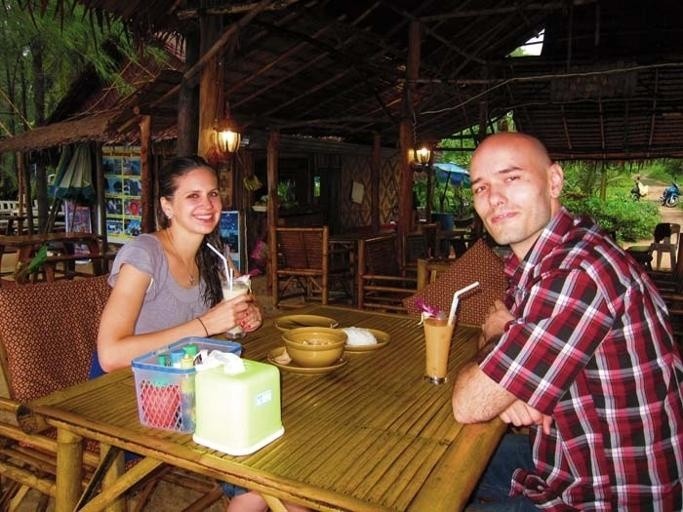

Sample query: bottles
[157,344,200,434]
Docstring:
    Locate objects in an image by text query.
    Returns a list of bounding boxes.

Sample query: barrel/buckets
[431,212,454,257]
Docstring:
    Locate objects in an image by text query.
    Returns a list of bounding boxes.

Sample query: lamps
[414,141,432,165]
[213,99,241,160]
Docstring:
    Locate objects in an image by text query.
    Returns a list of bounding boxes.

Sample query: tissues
[192,350,286,457]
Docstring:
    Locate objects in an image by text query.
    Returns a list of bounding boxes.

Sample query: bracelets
[196,317,208,337]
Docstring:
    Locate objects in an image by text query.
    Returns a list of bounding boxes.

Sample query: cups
[421,315,457,385]
[219,276,251,340]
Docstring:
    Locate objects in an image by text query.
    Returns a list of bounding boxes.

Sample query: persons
[453,133,683,512]
[51,143,96,207]
[88,154,317,510]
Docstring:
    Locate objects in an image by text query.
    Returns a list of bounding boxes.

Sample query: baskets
[131,337,242,434]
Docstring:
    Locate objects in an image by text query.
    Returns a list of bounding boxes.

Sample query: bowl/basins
[271,314,340,331]
[281,326,346,367]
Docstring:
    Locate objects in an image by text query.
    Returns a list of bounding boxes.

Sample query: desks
[25,306,509,511]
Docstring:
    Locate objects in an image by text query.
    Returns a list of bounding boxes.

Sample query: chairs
[275,217,512,327]
[653,222,680,271]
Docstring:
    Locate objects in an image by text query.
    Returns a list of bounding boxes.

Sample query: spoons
[274,343,291,366]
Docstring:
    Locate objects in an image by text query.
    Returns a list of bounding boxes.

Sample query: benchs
[0,276,176,511]
[26,252,117,282]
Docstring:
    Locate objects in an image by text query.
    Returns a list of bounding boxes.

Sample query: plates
[265,346,348,377]
[337,327,391,353]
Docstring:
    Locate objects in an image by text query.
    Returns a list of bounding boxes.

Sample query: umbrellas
[415,162,471,204]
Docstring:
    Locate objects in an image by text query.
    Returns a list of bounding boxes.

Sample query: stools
[626,246,654,270]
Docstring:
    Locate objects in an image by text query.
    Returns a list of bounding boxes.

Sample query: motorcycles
[630,176,642,202]
[658,176,681,209]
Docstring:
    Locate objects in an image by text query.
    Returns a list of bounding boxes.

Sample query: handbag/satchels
[249,238,267,268]
